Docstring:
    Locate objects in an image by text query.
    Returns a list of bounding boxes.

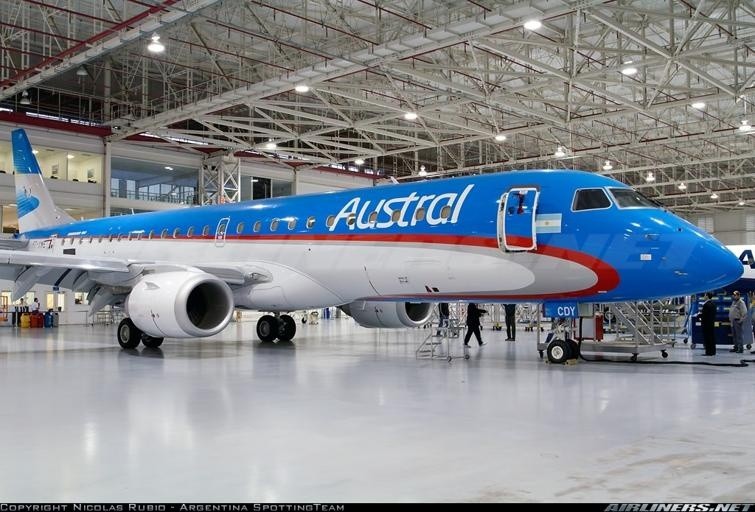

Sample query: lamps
[147,30,166,53]
[265,19,751,208]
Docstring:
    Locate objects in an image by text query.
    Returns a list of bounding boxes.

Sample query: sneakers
[463,342,486,348]
[730,349,743,352]
[505,337,515,341]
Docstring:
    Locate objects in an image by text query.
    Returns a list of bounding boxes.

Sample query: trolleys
[535,298,680,362]
[681,288,754,351]
[414,302,545,364]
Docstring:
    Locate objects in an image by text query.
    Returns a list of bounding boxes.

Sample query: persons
[701,291,716,356]
[728,289,748,354]
[18,298,27,306]
[436,303,457,337]
[750,291,755,355]
[31,298,40,312]
[463,303,488,348]
[502,304,516,341]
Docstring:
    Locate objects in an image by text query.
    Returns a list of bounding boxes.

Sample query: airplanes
[0,126,746,364]
